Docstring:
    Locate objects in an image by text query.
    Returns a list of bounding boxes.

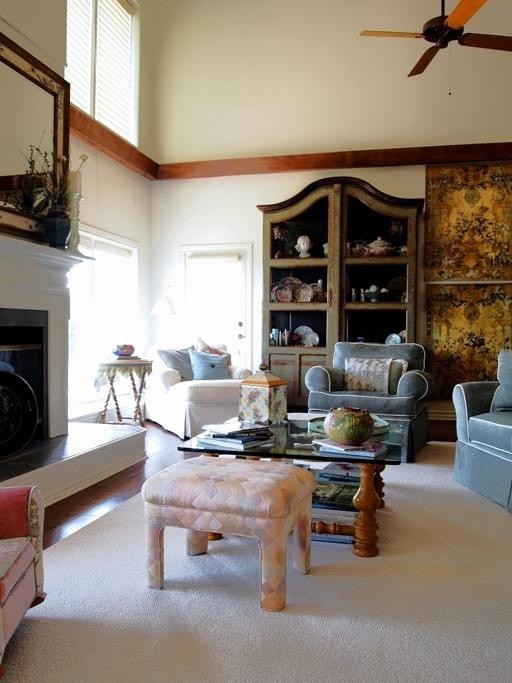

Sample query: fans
[355,0,512,78]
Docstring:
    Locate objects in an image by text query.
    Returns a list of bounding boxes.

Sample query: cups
[283,330,292,346]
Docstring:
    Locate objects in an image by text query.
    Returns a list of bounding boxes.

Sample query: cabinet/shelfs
[258,175,428,419]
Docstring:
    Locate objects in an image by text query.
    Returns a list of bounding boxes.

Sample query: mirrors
[0,29,73,241]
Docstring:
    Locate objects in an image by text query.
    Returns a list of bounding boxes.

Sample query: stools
[141,452,317,612]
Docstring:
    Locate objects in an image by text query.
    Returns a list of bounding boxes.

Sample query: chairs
[301,340,439,468]
[145,343,253,440]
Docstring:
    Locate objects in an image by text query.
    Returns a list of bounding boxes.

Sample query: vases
[44,203,72,250]
[323,406,375,446]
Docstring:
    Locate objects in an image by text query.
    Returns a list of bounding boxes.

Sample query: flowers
[33,140,91,207]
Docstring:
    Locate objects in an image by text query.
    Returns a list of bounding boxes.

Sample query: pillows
[488,345,512,412]
[156,337,233,381]
[342,354,409,395]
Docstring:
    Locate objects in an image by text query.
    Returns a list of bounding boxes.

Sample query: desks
[95,360,154,426]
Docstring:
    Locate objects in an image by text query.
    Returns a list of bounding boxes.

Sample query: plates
[294,324,320,346]
[270,276,313,302]
[385,333,402,344]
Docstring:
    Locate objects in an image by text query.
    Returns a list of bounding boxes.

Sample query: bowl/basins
[367,236,394,255]
[364,290,383,303]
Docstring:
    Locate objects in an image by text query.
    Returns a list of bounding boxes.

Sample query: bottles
[271,328,280,346]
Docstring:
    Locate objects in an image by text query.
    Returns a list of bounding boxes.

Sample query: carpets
[2,438,512,682]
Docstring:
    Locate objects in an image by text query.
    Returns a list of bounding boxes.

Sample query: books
[198,417,275,450]
[312,462,361,507]
[311,435,387,456]
